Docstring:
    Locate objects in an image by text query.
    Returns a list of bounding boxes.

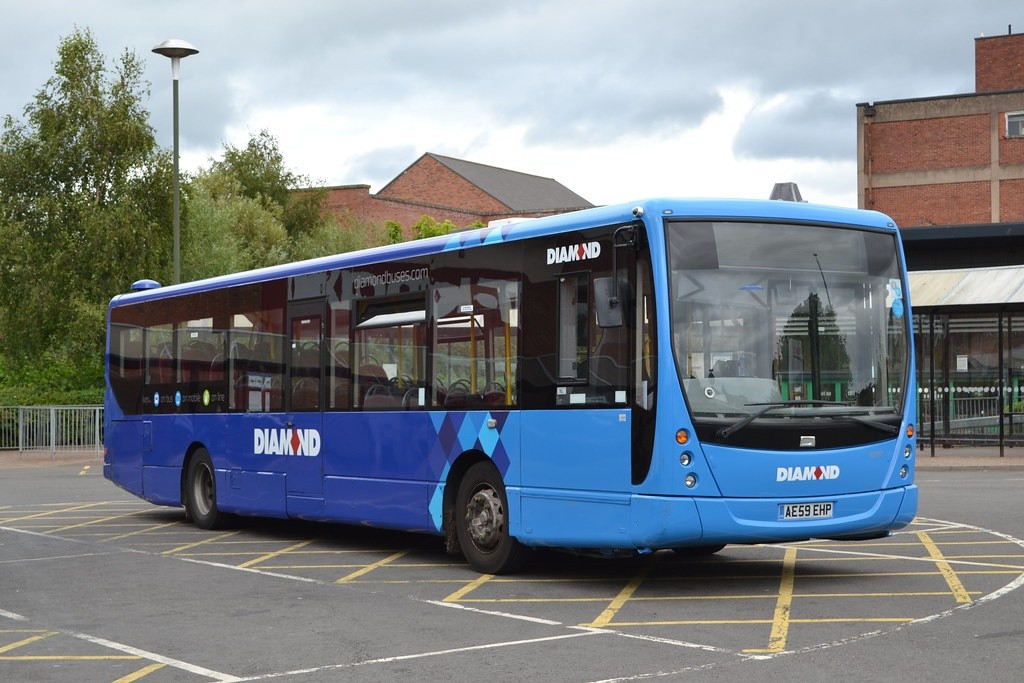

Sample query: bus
[102,197,919,575]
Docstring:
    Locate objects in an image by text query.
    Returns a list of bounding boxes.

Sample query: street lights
[151,39,200,286]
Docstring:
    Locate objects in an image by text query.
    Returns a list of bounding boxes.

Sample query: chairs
[125,340,514,411]
